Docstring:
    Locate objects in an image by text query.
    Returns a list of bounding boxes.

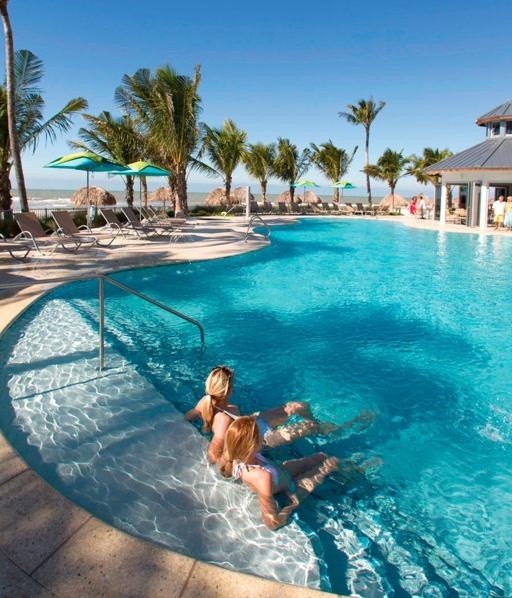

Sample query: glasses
[211,365,235,391]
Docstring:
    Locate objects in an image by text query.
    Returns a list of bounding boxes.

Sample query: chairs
[217,197,404,215]
[2,198,196,260]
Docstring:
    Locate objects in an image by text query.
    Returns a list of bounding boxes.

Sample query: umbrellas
[44,149,131,238]
[329,180,356,215]
[108,159,172,233]
[287,180,320,214]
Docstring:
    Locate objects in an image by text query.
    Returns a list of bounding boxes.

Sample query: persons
[492,194,505,231]
[418,196,424,215]
[503,196,511,231]
[215,415,385,532]
[332,190,337,202]
[182,365,378,467]
[409,196,417,215]
